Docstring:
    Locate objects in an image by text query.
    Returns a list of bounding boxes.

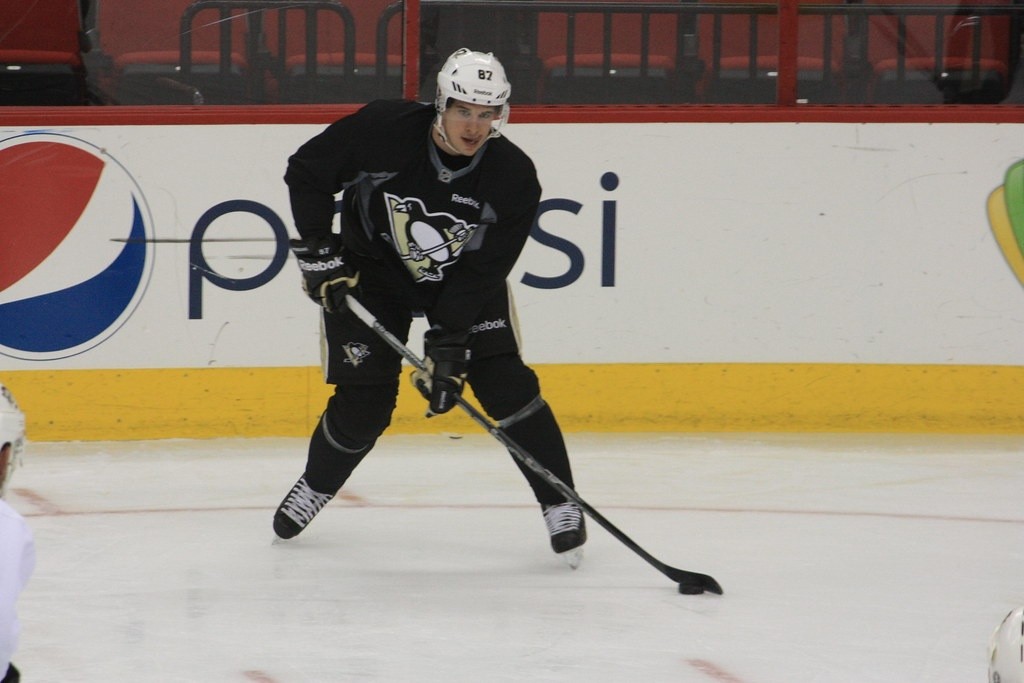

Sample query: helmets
[435,46,512,116]
[988,607,1024,683]
[0,385,26,452]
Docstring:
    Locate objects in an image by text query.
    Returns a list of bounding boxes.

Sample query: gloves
[410,326,476,418]
[287,238,365,316]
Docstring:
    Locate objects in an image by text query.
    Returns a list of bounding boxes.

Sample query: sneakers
[541,497,588,570]
[271,472,333,543]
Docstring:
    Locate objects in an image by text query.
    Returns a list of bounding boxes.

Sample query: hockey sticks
[343,292,728,597]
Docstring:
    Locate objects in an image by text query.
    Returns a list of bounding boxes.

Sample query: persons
[270,46,589,569]
[0,392,35,683]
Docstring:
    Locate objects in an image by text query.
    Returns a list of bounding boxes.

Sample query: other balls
[679,582,704,595]
